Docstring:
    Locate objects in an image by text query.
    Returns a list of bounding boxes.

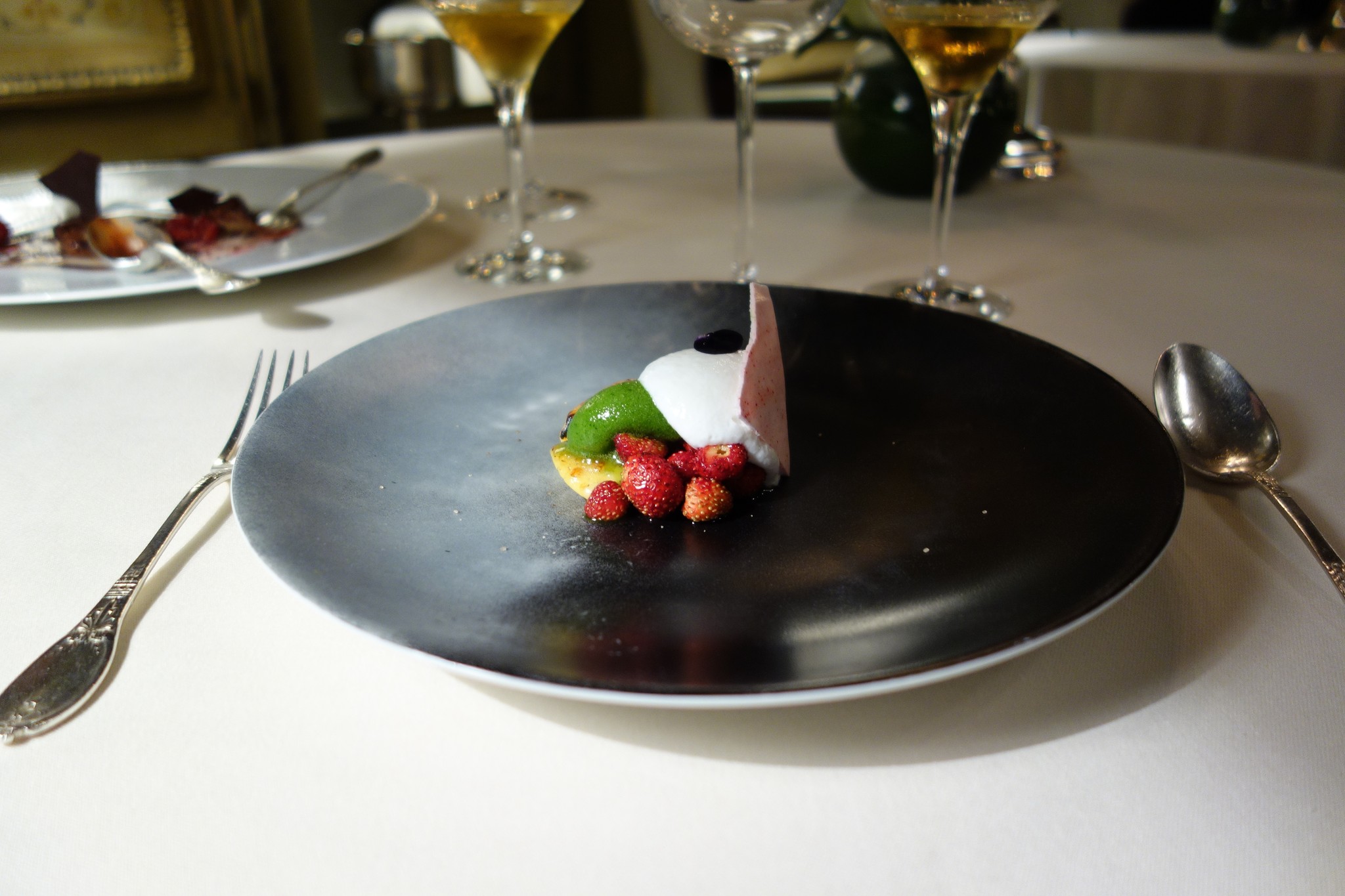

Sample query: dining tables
[0,111,1345,896]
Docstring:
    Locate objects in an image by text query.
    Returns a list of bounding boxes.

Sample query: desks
[1010,27,1345,170]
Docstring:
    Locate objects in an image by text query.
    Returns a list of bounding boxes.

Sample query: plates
[1,166,437,304]
[232,278,1188,711]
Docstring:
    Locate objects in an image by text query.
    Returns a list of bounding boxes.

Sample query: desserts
[160,184,260,244]
[551,283,790,500]
[40,149,144,256]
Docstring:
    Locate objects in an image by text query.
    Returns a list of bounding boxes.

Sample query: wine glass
[654,0,843,284]
[862,1,1061,325]
[423,1,595,284]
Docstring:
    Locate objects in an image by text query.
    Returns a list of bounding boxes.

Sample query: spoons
[86,219,257,296]
[1151,342,1344,599]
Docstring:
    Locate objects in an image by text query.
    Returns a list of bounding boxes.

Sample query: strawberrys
[582,444,746,522]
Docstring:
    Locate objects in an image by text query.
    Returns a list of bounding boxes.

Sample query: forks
[1,349,312,746]
